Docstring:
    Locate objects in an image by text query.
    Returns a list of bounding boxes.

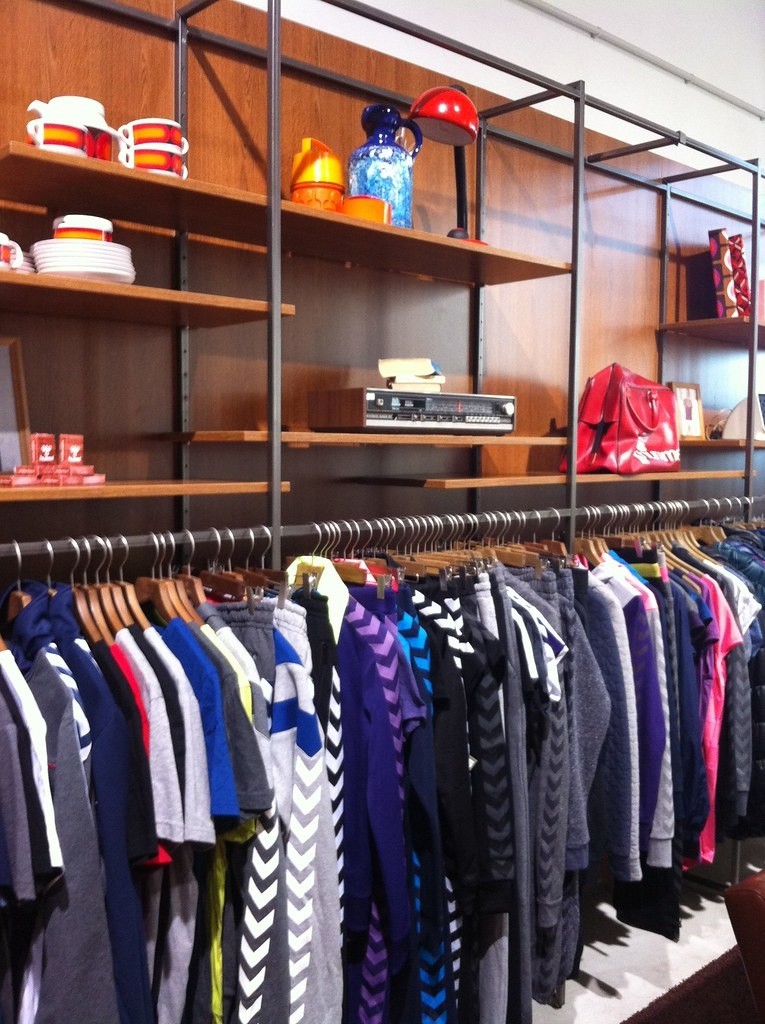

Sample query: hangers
[1,493,764,655]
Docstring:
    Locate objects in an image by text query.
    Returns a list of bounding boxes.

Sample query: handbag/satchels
[559,362,682,474]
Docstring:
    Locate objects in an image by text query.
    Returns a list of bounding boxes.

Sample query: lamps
[406,85,489,246]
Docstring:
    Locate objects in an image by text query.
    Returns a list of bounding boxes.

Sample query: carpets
[619,944,761,1023]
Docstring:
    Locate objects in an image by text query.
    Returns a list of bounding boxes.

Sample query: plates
[12,239,138,285]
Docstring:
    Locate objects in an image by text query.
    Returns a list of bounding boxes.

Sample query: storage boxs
[0,431,105,489]
[708,227,739,318]
[727,233,752,316]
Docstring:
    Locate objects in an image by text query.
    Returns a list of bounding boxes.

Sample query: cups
[120,118,189,153]
[123,144,190,179]
[51,211,115,240]
[0,232,23,268]
[343,195,393,225]
[27,118,88,159]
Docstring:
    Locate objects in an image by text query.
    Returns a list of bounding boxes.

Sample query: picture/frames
[668,381,707,441]
[0,335,33,474]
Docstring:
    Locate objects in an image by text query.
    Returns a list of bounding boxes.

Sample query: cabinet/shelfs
[0,0,765,501]
